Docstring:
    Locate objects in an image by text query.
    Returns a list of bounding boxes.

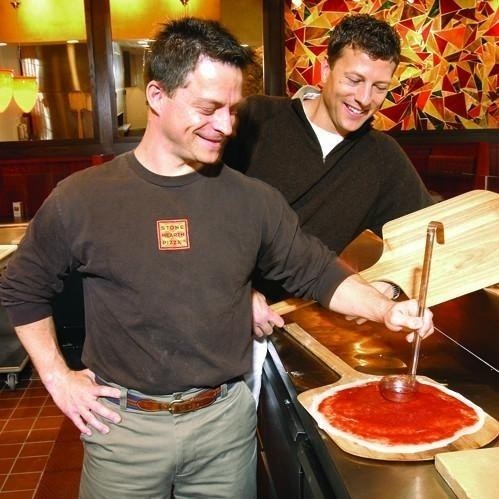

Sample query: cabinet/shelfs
[258,339,351,499]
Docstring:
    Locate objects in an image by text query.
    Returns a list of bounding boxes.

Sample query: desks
[0,216,34,244]
[0,244,23,270]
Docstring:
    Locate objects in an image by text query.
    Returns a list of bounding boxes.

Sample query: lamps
[12,2,37,112]
[0,69,14,112]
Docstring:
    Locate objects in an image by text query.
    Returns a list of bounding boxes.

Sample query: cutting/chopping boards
[285,322,499,461]
[270,188,499,316]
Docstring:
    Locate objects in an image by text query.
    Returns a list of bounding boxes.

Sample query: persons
[0,16,434,499]
[228,15,433,414]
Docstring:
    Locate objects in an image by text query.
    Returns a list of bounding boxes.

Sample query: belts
[92,373,245,416]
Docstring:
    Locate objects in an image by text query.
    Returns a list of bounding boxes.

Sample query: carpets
[35,416,85,499]
